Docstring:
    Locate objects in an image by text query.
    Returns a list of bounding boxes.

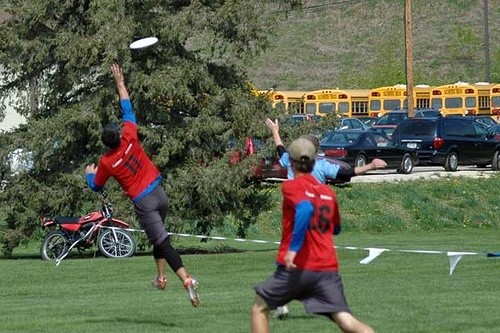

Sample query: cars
[212,136,286,185]
[331,117,367,131]
[320,130,415,173]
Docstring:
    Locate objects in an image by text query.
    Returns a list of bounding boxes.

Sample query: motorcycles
[40,189,135,260]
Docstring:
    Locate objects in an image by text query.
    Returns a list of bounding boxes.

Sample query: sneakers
[153,276,167,290]
[274,304,289,318]
[183,275,200,308]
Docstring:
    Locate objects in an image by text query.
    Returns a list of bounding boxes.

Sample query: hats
[289,138,316,162]
[301,135,320,148]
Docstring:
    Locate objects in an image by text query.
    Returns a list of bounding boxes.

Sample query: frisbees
[129,37,158,49]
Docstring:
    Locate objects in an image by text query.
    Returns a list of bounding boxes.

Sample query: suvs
[372,108,442,139]
[392,116,500,170]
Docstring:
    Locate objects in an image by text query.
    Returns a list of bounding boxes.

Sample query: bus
[490,84,500,123]
[256,90,307,114]
[430,84,493,115]
[368,86,433,117]
[304,89,371,118]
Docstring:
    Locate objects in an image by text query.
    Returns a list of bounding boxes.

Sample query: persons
[251,138,375,332]
[265,118,387,320]
[85,63,200,308]
